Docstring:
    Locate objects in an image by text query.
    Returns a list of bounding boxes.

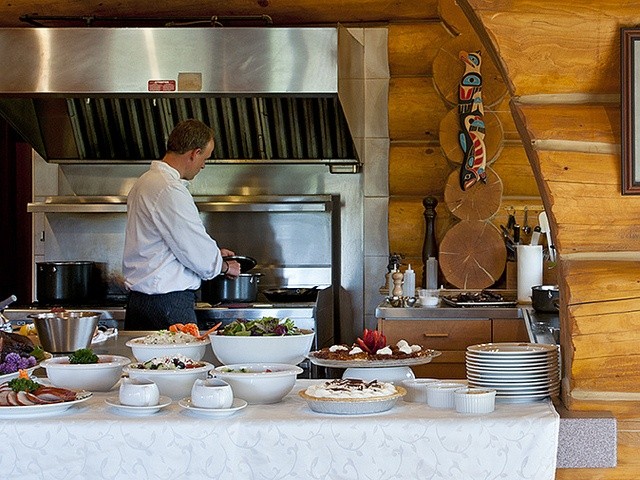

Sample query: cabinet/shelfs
[378,318,531,378]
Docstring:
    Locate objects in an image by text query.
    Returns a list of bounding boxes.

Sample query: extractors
[0,25,362,175]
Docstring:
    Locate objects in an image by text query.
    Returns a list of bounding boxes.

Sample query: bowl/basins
[26,312,102,353]
[403,378,440,402]
[121,361,214,396]
[191,378,233,408]
[118,376,159,406]
[209,363,303,405]
[426,383,465,409]
[418,289,440,306]
[207,329,315,365]
[40,355,131,392]
[453,387,497,415]
[125,336,211,363]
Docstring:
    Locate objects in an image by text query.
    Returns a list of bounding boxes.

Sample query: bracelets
[223,261,230,274]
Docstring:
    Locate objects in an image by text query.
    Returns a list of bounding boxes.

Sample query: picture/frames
[620,26,640,194]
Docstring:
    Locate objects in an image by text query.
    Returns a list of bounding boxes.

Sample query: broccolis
[68,349,98,364]
[8,378,40,392]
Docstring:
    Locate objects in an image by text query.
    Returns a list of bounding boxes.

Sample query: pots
[214,273,262,302]
[263,288,318,301]
[532,285,559,312]
[36,260,96,306]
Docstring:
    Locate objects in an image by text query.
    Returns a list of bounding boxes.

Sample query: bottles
[388,264,398,297]
[392,270,403,296]
[426,256,439,289]
[403,263,415,297]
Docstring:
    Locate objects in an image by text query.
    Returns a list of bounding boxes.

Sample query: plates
[0,351,53,383]
[105,396,173,414]
[177,396,247,416]
[299,386,407,415]
[0,390,94,414]
[303,347,442,368]
[465,342,561,402]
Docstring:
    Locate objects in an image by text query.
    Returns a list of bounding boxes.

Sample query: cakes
[306,380,396,398]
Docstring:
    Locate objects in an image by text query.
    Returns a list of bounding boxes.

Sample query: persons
[122,120,241,331]
